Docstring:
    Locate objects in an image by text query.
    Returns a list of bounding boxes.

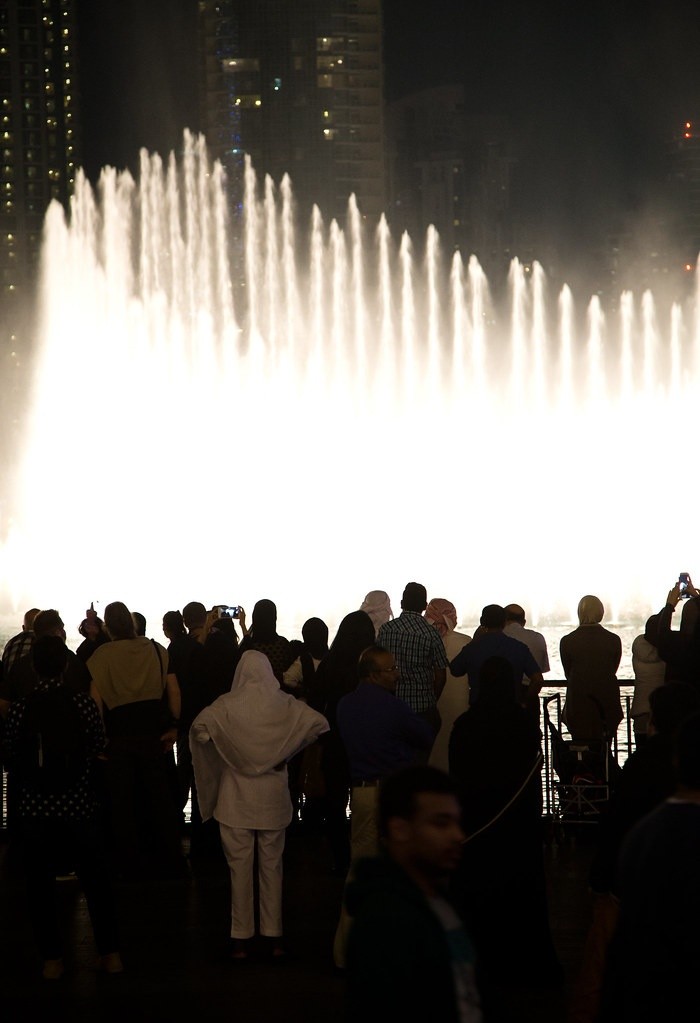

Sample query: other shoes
[263,946,284,960]
[232,945,251,964]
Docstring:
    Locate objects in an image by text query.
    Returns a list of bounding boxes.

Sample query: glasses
[373,664,401,674]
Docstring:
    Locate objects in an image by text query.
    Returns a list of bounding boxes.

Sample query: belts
[351,777,384,788]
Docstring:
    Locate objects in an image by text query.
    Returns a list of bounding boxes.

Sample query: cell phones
[218,607,240,618]
[679,573,691,598]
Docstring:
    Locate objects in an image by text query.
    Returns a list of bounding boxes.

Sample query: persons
[0,574,700,1022]
